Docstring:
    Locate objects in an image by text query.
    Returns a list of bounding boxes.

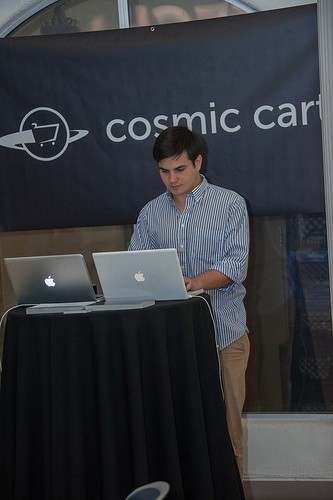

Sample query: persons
[125,126,252,485]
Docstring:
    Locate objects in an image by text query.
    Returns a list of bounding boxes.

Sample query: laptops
[4,254,104,304]
[92,249,205,300]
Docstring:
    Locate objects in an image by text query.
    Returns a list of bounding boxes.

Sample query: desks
[0,292,246,499]
